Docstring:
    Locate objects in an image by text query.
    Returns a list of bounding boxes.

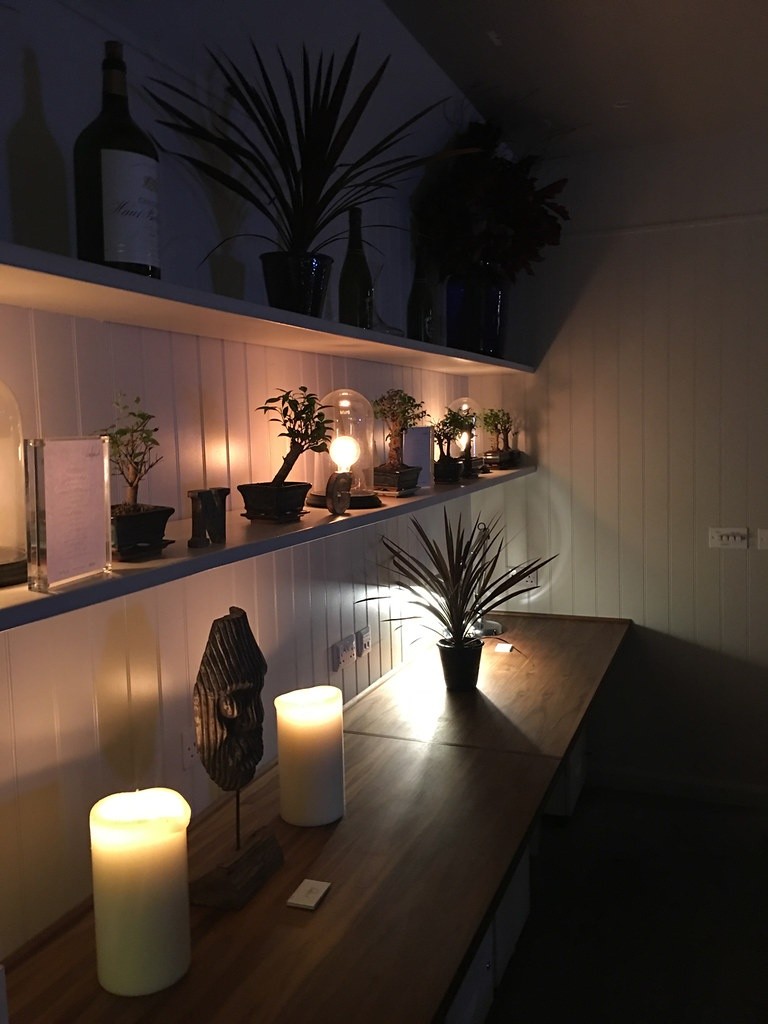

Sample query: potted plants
[434,150,573,359]
[352,505,562,692]
[432,406,525,477]
[142,29,485,319]
[92,391,175,551]
[368,388,432,492]
[238,384,335,517]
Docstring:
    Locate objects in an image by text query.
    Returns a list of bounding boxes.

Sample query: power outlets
[519,567,539,588]
[331,633,358,672]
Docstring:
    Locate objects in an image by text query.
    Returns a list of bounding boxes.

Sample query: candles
[88,784,193,998]
[273,685,345,828]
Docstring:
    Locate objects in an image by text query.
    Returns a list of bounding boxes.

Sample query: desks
[1,609,634,1024]
